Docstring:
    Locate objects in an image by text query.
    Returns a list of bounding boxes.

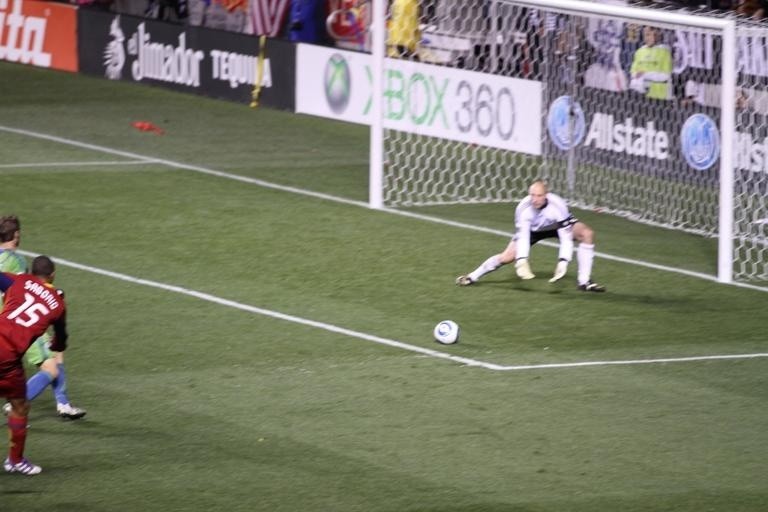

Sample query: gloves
[548,261,569,282]
[514,258,536,280]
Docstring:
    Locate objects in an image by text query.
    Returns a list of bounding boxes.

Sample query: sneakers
[456,275,473,286]
[57,405,86,419]
[576,282,605,291]
[3,457,43,475]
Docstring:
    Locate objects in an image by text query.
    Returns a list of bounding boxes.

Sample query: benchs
[104,0,298,41]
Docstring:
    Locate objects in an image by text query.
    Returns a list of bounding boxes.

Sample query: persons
[387,1,766,104]
[1,215,86,421]
[1,255,68,476]
[456,180,606,294]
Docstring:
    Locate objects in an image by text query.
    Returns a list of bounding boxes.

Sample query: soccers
[433,319,458,344]
